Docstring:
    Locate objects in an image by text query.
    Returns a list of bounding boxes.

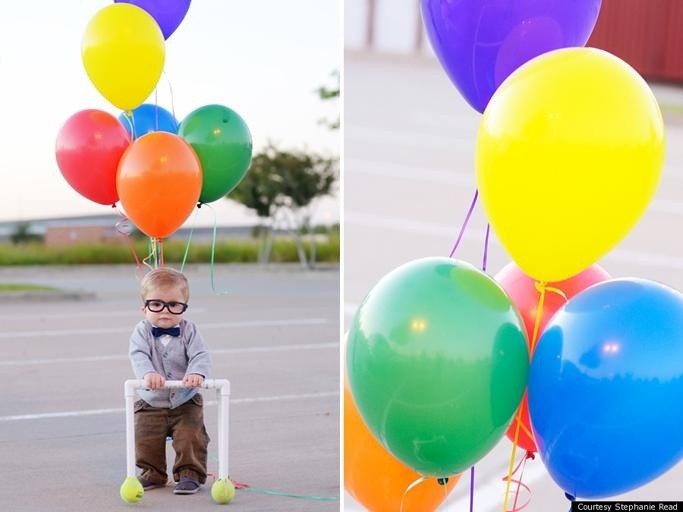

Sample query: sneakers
[134,472,165,491]
[172,475,202,494]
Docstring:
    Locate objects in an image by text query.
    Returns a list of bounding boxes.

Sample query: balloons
[528,277,683,511]
[55,109,131,207]
[118,104,179,142]
[179,104,253,209]
[113,0,191,40]
[496,262,609,458]
[476,47,665,295]
[344,369,461,512]
[80,3,167,115]
[419,0,602,112]
[345,255,530,487]
[115,131,203,243]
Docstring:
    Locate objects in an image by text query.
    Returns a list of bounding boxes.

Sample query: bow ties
[151,327,181,337]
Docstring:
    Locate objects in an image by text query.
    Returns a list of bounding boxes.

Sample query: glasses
[143,299,188,315]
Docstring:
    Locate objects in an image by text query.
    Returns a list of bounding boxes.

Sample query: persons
[128,267,211,494]
